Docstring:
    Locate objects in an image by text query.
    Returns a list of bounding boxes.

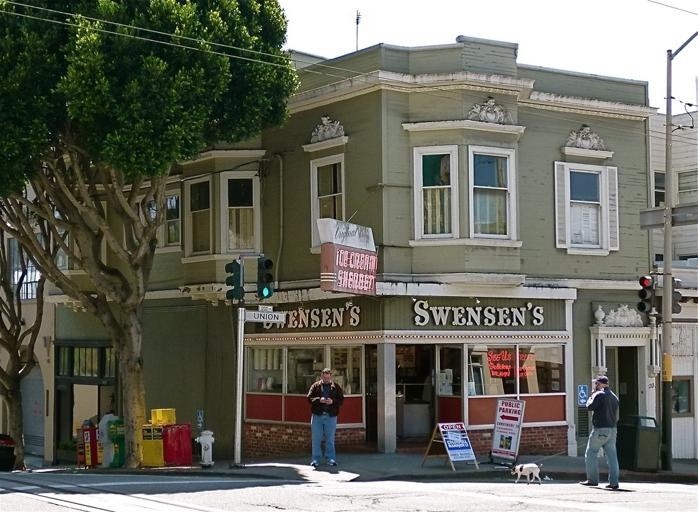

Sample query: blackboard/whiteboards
[438,423,476,461]
[491,398,526,459]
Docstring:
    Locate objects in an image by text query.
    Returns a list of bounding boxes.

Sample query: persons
[98,409,119,469]
[307,367,344,468]
[579,375,620,489]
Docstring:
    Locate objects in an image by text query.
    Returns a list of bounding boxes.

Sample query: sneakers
[310,460,320,468]
[326,458,337,466]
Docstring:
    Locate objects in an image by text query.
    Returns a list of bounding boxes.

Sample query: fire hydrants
[195,430,215,467]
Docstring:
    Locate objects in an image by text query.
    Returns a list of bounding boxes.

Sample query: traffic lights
[258,255,274,301]
[224,257,245,300]
[637,275,653,313]
[672,277,682,314]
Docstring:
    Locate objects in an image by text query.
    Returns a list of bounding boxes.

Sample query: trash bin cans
[616,415,660,472]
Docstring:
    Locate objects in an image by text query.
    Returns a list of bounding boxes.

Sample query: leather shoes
[579,479,598,486]
[605,484,618,489]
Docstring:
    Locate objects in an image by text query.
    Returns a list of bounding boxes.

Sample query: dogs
[510,463,543,484]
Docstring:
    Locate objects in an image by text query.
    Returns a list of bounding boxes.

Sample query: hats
[592,375,608,384]
